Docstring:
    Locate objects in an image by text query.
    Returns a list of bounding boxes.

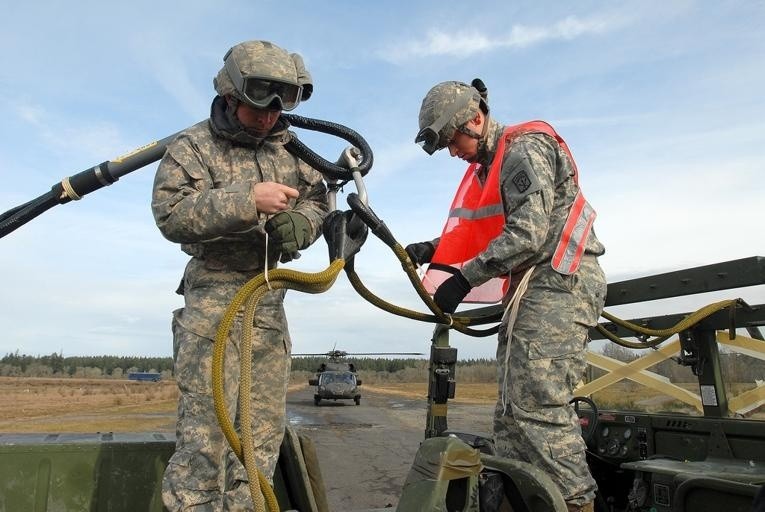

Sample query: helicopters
[289,341,426,404]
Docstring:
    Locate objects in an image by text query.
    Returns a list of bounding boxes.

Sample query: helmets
[417,81,488,148]
[213,40,299,111]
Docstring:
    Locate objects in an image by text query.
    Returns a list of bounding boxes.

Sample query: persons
[150,40,333,511]
[396,77,606,511]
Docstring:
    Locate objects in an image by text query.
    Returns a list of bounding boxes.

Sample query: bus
[128,372,161,382]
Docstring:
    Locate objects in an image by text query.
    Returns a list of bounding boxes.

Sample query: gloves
[264,211,311,255]
[405,242,434,269]
[433,270,472,314]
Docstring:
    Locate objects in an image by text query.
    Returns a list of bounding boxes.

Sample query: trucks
[0,257,765,510]
